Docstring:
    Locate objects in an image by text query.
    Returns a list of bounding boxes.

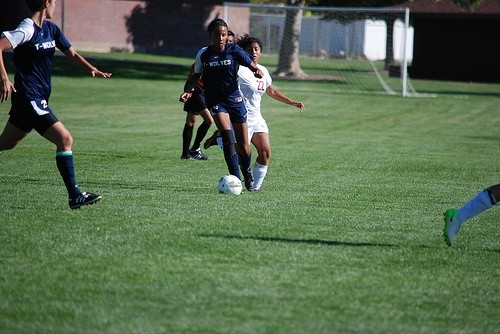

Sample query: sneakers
[442,208,460,248]
[240,164,255,192]
[68,191,102,211]
[187,149,209,161]
[204,130,222,150]
[180,152,193,159]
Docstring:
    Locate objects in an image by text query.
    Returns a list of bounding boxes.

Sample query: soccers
[218,175,243,195]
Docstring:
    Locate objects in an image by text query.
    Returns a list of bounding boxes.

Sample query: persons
[181,31,235,160]
[179,19,263,194]
[0,0,112,209]
[442,184,500,247]
[204,34,304,191]
